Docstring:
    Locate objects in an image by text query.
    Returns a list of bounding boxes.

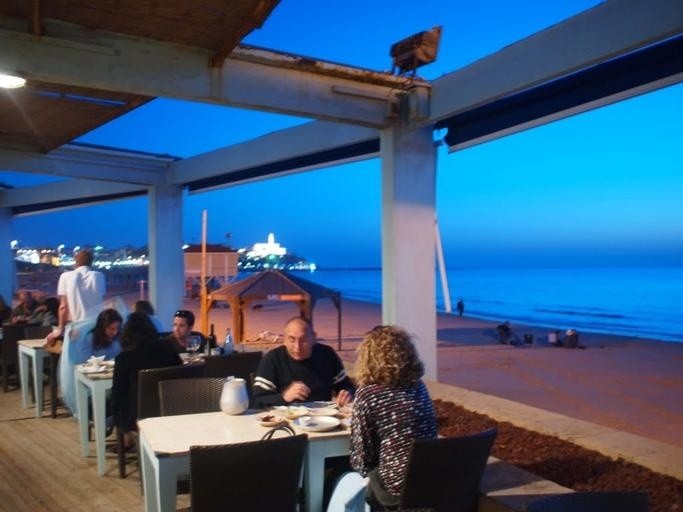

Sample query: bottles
[190,335,200,362]
[206,323,218,355]
[223,327,233,354]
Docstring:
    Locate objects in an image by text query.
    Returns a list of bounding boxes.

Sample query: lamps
[390,26,441,74]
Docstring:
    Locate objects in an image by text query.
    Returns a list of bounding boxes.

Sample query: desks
[136,400,352,510]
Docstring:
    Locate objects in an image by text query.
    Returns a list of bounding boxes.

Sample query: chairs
[180,432,308,511]
[366,429,497,510]
[3,310,263,494]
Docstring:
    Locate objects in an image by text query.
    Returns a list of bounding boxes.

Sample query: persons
[164,310,209,354]
[328,325,439,511]
[133,300,164,332]
[11,289,40,322]
[66,307,124,437]
[25,297,59,327]
[46,251,108,424]
[456,298,465,315]
[250,316,357,408]
[103,311,180,454]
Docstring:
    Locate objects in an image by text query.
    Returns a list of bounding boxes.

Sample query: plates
[254,413,286,427]
[299,416,340,431]
[305,406,340,416]
[77,362,108,374]
[275,408,307,419]
[341,416,364,429]
